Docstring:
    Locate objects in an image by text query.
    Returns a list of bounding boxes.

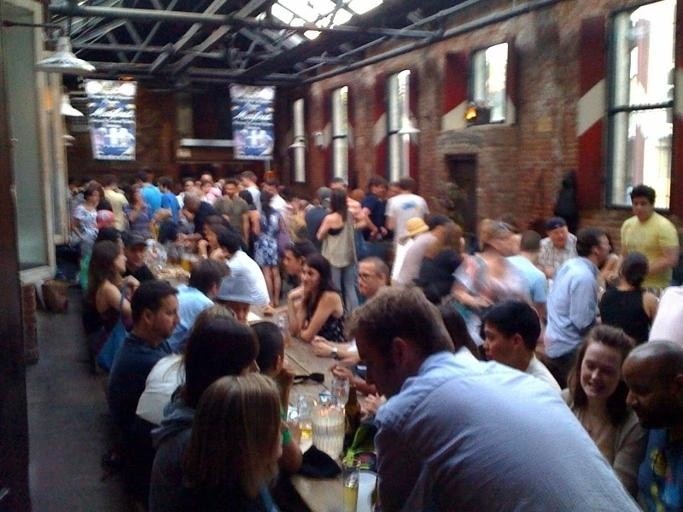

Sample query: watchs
[330,345,339,358]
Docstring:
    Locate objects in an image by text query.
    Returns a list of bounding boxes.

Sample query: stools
[84,332,108,376]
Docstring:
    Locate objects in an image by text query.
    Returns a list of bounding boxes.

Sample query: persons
[200,172,213,183]
[177,177,195,208]
[481,299,561,402]
[543,227,612,364]
[599,249,659,347]
[542,218,580,273]
[287,252,343,346]
[622,339,683,512]
[79,226,122,292]
[387,170,431,232]
[277,192,299,252]
[333,278,430,394]
[312,258,394,358]
[251,320,302,469]
[478,218,503,252]
[177,191,215,243]
[150,209,172,237]
[158,176,180,225]
[198,224,230,265]
[363,302,469,417]
[157,220,179,260]
[264,177,284,224]
[200,181,216,206]
[319,187,359,311]
[175,374,290,512]
[620,184,680,291]
[164,258,235,356]
[417,223,467,306]
[218,232,270,308]
[306,187,332,238]
[84,242,140,345]
[252,192,282,308]
[131,307,238,426]
[387,180,400,199]
[146,314,258,512]
[395,216,452,287]
[121,231,155,283]
[238,190,261,257]
[204,215,223,232]
[507,225,549,314]
[361,175,388,259]
[350,188,365,202]
[649,283,683,342]
[67,169,160,248]
[240,171,263,213]
[214,181,250,245]
[391,217,430,281]
[329,177,370,231]
[561,322,642,494]
[451,219,539,358]
[343,285,643,512]
[262,237,320,318]
[105,281,180,424]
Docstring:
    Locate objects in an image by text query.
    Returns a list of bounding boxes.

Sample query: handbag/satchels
[352,215,368,262]
[94,281,131,375]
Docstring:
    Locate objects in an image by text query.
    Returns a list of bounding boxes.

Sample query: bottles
[312,389,344,479]
[344,385,361,451]
[329,379,344,413]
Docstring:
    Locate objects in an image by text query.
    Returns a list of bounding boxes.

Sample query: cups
[289,395,309,420]
[332,377,349,408]
[314,405,345,462]
[300,403,313,441]
[342,459,361,508]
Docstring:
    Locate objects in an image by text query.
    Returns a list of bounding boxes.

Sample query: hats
[404,217,429,239]
[122,229,148,250]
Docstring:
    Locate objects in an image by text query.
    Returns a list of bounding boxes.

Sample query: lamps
[60,94,83,116]
[3,19,95,74]
